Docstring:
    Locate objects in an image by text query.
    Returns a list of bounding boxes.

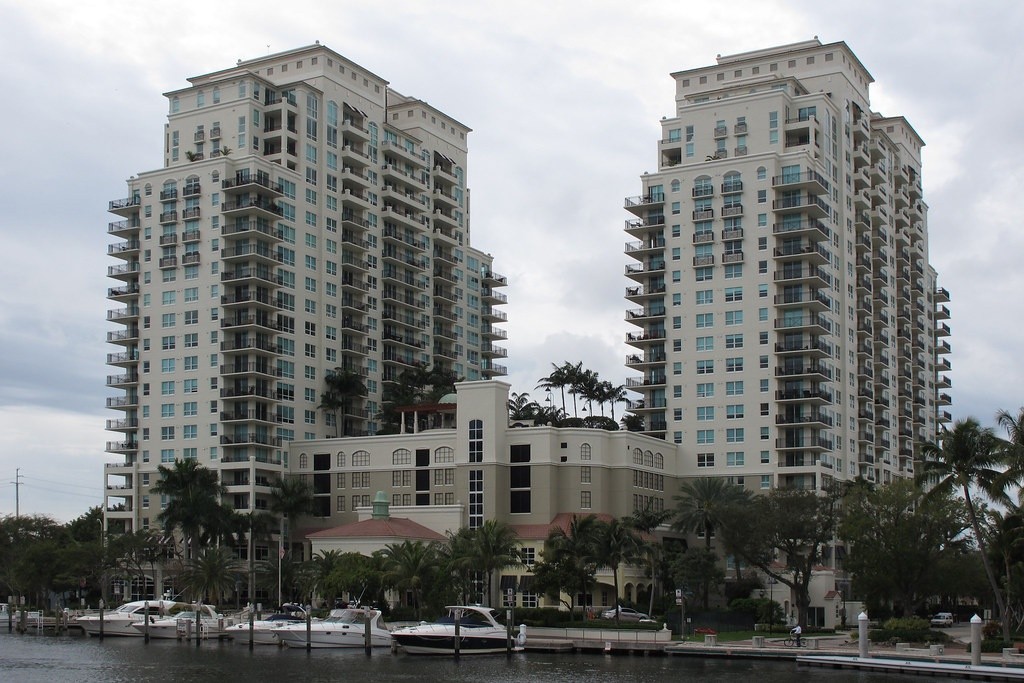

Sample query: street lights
[97,519,103,547]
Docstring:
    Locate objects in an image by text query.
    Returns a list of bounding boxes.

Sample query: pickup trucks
[931,613,953,628]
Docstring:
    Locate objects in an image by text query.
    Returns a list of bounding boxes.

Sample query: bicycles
[784,631,807,647]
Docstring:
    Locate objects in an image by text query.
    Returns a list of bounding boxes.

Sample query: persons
[792,624,801,647]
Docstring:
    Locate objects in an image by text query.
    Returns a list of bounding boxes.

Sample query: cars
[601,608,649,622]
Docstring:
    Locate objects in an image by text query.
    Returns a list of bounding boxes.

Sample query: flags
[280,543,285,559]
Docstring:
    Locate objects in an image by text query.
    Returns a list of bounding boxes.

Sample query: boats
[75,601,174,637]
[269,605,401,647]
[224,605,320,645]
[132,603,234,639]
[391,605,525,657]
